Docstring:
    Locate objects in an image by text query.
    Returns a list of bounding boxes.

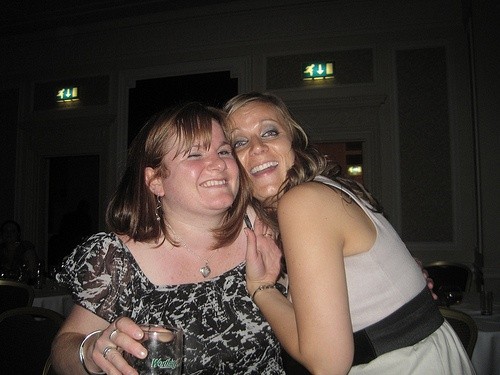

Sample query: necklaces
[164,218,211,278]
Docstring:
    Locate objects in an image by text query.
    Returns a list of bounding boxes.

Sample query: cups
[122,323,183,375]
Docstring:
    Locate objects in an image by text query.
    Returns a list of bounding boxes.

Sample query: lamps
[54,86,81,103]
[301,62,335,79]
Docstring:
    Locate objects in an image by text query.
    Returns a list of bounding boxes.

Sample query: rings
[109,330,116,339]
[103,346,112,358]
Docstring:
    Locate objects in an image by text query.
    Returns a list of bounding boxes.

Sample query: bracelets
[79,330,107,375]
[253,285,276,300]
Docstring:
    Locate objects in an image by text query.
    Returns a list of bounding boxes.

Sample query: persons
[221,91,478,375]
[50,103,437,375]
